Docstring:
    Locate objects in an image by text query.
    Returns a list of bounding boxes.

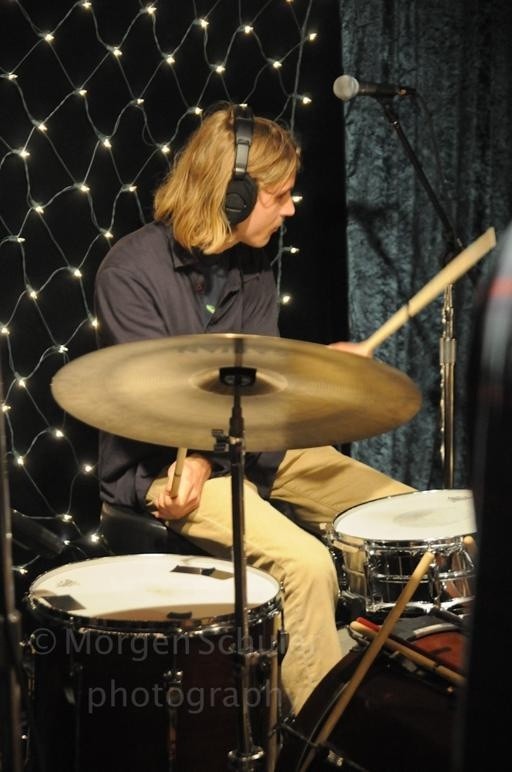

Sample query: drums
[22,551,290,772]
[274,608,472,772]
[320,487,478,630]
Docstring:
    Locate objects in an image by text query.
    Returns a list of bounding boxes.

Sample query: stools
[98,498,292,555]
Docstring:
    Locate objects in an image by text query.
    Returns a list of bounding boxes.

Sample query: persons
[93,107,478,721]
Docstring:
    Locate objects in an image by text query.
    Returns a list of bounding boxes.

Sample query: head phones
[222,101,256,225]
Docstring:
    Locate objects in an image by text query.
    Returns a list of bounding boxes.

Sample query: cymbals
[50,332,422,452]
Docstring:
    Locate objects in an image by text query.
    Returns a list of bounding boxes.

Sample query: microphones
[331,74,414,103]
